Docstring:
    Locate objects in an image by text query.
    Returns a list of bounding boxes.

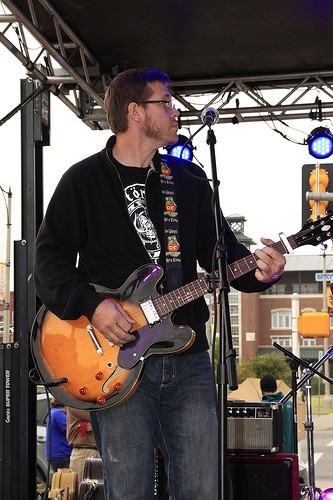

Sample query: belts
[75,446,98,451]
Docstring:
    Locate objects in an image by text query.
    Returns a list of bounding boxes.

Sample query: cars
[36,393,55,495]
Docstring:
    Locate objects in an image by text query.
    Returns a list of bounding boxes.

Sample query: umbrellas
[228,378,306,444]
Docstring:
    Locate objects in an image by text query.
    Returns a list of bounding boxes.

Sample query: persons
[66,402,101,482]
[33,68,286,500]
[46,397,71,472]
[259,375,298,454]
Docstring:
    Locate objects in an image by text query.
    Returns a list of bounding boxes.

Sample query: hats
[260,375,277,392]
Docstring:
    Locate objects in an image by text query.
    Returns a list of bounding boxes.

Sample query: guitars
[29,210,332,412]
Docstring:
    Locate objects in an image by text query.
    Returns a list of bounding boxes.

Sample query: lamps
[304,96,333,158]
[162,108,197,161]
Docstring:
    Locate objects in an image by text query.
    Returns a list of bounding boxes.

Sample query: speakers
[157,452,299,500]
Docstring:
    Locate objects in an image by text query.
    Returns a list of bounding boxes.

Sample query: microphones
[200,107,219,124]
[28,368,69,387]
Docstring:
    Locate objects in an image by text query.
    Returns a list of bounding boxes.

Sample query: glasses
[134,99,176,110]
